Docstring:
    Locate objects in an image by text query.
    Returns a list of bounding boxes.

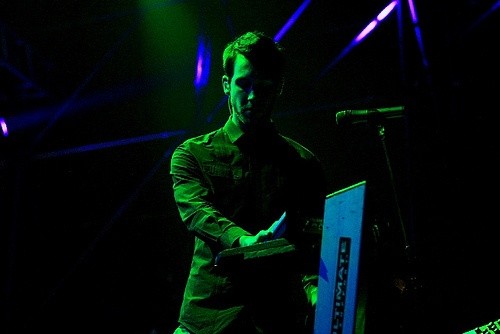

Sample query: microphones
[336,105,407,125]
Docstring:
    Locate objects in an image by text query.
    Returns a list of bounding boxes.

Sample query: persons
[169,30,325,334]
